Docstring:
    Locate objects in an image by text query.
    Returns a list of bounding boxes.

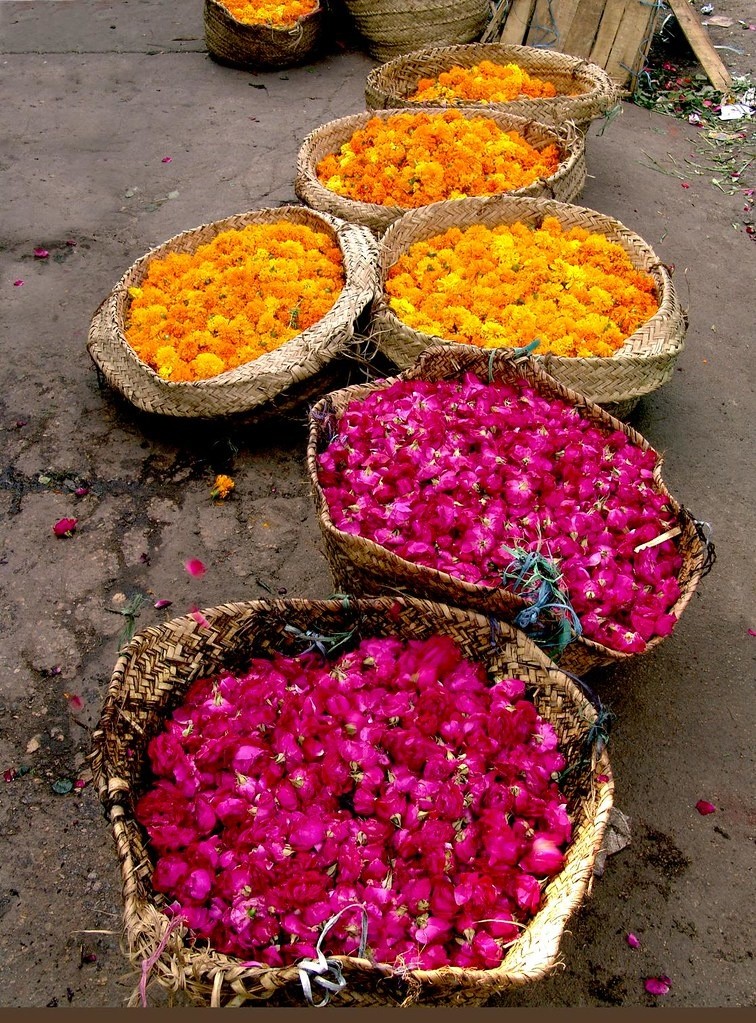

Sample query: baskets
[97,592,614,1008]
[204,0,334,77]
[87,33,688,426]
[344,0,494,63]
[306,342,709,684]
